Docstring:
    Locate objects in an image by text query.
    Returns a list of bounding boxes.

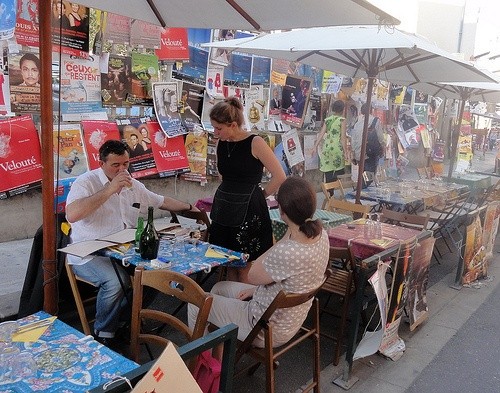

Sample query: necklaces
[227,142,236,157]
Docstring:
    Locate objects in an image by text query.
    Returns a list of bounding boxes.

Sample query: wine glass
[364,213,376,240]
[0,320,23,385]
[374,211,383,240]
[188,225,202,252]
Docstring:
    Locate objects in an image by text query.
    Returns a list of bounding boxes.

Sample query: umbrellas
[200,25,498,203]
[408,57,500,182]
[39,0,400,317]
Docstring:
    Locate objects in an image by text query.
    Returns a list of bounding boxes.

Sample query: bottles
[135,217,144,249]
[139,207,160,260]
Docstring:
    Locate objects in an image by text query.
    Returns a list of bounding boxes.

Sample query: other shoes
[93,333,125,356]
[115,328,130,344]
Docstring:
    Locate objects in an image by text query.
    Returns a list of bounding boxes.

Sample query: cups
[398,180,413,198]
[11,352,38,384]
[417,178,434,190]
[377,182,391,201]
[120,169,134,193]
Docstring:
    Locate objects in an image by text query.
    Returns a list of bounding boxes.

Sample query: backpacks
[365,117,383,158]
[191,350,221,393]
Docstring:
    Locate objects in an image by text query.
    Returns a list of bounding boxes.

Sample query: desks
[329,218,421,331]
[0,310,141,393]
[345,185,452,265]
[400,178,470,254]
[269,207,353,247]
[441,173,491,213]
[91,238,247,361]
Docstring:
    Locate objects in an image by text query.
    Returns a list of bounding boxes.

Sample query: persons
[65,139,207,351]
[122,127,152,156]
[352,102,386,190]
[19,53,41,88]
[56,2,85,30]
[270,88,281,110]
[208,97,287,282]
[107,71,135,103]
[310,101,351,198]
[286,92,299,117]
[180,90,190,121]
[188,176,330,349]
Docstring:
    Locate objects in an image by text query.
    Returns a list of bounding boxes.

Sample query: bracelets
[189,203,193,211]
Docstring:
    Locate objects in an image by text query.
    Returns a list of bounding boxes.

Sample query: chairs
[65,162,500,393]
